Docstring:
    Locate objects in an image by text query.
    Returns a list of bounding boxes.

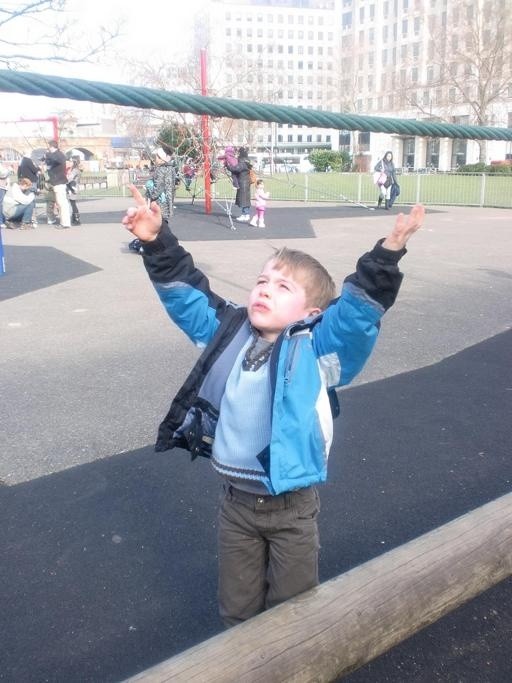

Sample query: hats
[158,147,173,162]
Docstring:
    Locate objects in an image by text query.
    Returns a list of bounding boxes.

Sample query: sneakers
[7,222,35,230]
[48,213,81,228]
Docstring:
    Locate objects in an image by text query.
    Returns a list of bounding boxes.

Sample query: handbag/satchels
[373,172,392,188]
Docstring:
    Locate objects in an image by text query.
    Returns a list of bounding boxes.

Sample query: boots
[236,214,265,228]
[377,198,390,210]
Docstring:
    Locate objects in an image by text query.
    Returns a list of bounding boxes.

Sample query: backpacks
[249,170,256,183]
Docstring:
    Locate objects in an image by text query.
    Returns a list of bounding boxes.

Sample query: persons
[184,158,195,192]
[249,180,271,227]
[1,139,91,231]
[226,146,253,221]
[375,152,398,207]
[124,181,426,629]
[225,145,239,189]
[139,148,177,254]
